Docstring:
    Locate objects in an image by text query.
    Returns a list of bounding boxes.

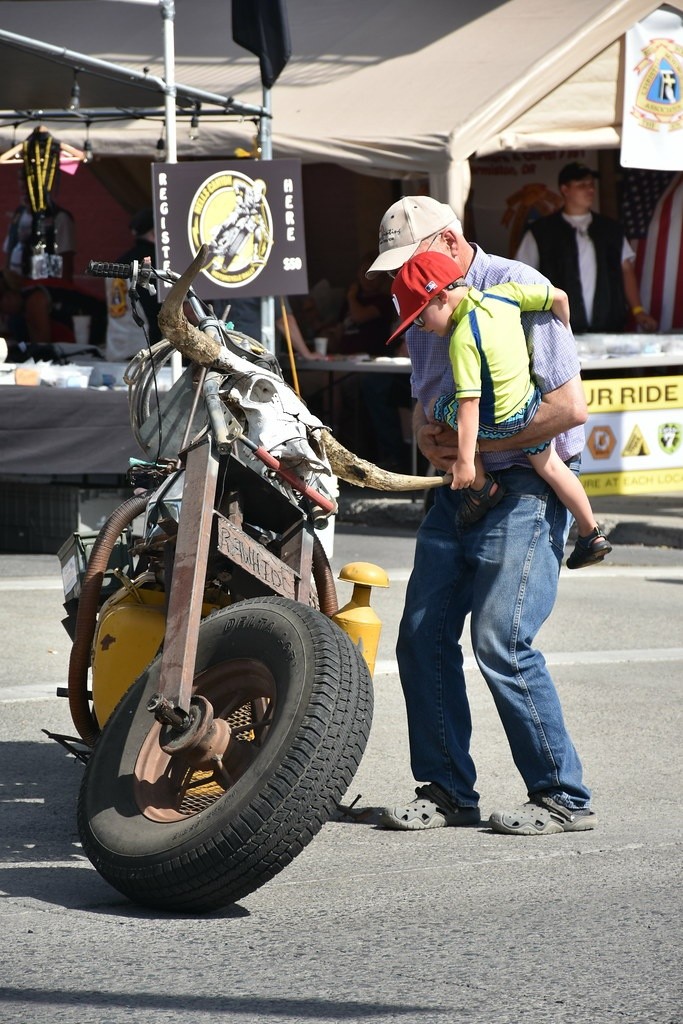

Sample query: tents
[0,0,683,555]
[1,28,317,555]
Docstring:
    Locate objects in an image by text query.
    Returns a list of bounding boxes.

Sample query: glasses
[414,295,439,328]
[387,266,403,280]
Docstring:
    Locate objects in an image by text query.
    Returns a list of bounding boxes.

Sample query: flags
[614,158,683,334]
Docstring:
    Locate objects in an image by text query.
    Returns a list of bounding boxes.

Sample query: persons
[392,252,613,570]
[120,203,164,346]
[278,246,412,473]
[514,162,659,335]
[376,195,596,836]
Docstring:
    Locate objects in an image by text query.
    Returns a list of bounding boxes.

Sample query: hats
[365,195,459,280]
[557,162,596,186]
[385,250,462,344]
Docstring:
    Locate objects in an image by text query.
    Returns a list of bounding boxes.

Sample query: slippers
[490,792,597,834]
[380,781,482,828]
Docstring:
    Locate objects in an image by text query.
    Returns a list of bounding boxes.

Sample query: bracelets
[632,305,644,316]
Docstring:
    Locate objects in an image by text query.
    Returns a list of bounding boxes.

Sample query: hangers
[0,109,87,165]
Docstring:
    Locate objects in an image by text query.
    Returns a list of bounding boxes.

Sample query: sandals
[458,472,504,528]
[567,526,612,569]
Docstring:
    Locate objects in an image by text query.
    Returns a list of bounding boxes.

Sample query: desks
[278,333,683,504]
[0,362,227,484]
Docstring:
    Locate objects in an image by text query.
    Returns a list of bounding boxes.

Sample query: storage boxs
[58,528,134,602]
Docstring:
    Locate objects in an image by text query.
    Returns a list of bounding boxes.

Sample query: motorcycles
[38,240,454,921]
